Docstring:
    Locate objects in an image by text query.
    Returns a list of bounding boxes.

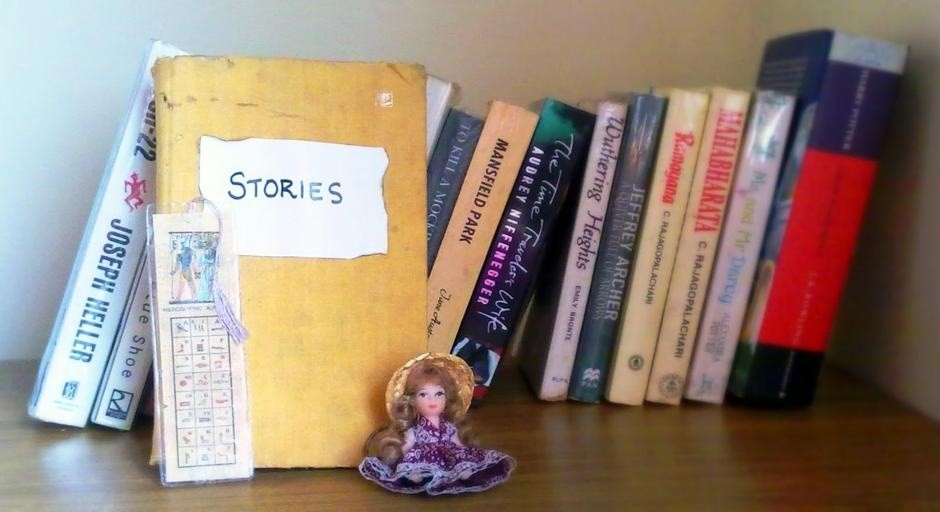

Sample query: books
[24,26,910,431]
[141,54,432,472]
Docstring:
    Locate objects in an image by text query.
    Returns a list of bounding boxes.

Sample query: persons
[359,352,516,496]
